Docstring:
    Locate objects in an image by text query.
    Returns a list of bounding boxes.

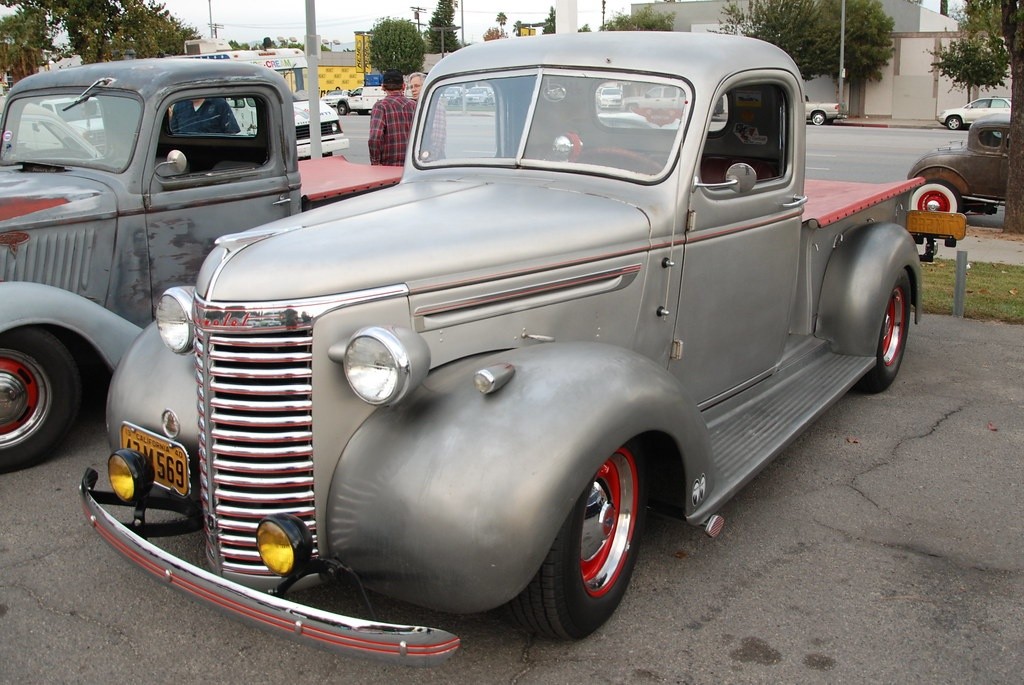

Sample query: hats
[383,69,404,84]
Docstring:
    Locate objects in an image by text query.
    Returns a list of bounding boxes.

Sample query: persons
[367,68,447,168]
[171,95,242,135]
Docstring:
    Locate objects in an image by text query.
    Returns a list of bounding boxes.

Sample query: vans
[337,86,386,116]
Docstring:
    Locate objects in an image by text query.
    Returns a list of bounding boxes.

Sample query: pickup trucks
[622,85,685,112]
[1,60,404,477]
[805,101,849,126]
[77,32,968,664]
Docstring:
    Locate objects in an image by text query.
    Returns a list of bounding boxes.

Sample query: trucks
[163,39,349,163]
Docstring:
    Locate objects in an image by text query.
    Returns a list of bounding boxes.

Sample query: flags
[519,27,537,36]
[354,33,372,73]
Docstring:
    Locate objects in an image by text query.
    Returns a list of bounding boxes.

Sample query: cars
[906,112,1011,214]
[938,97,1013,131]
[39,97,105,155]
[441,86,495,106]
[596,87,624,110]
[0,96,102,162]
[320,90,350,108]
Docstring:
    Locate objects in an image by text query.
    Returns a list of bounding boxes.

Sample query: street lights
[322,39,341,51]
[276,36,297,48]
[430,26,461,60]
